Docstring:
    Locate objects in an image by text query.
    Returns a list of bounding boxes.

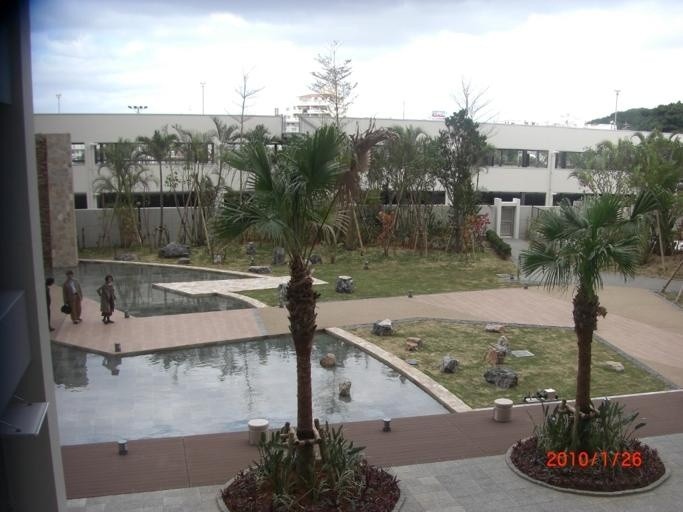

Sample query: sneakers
[73,318,81,324]
[102,319,113,324]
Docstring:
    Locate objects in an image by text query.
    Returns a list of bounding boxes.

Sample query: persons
[62,270,83,324]
[46,277,55,331]
[96,274,115,324]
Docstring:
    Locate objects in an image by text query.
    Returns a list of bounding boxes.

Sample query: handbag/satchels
[61,304,71,313]
